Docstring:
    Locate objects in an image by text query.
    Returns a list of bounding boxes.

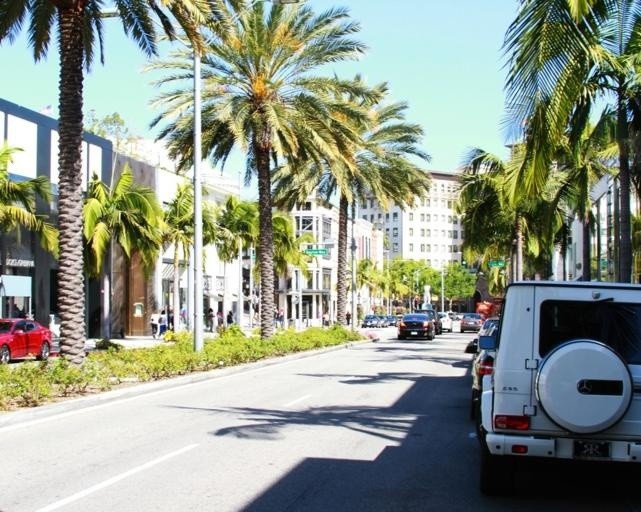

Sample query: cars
[461,312,498,424]
[0,318,51,365]
[362,309,461,341]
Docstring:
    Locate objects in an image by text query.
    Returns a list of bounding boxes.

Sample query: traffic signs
[304,249,326,255]
[487,260,504,266]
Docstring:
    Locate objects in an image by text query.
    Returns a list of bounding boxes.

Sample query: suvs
[481,278,640,502]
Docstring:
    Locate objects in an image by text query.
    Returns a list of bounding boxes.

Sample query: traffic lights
[460,252,466,265]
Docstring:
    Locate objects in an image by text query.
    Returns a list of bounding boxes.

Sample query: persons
[149,303,188,339]
[323,311,329,326]
[273,306,284,328]
[207,307,234,333]
[346,311,351,324]
